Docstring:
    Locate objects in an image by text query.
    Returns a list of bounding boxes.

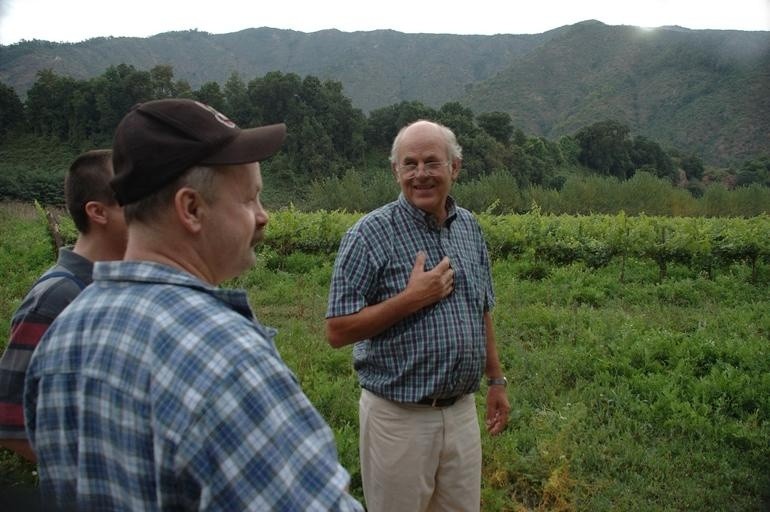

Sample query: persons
[327,119,514,512]
[0,147,130,467]
[21,98,369,512]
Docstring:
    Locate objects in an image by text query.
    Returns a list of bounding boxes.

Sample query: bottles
[109,100,289,196]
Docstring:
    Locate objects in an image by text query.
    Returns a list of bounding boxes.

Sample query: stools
[413,396,460,412]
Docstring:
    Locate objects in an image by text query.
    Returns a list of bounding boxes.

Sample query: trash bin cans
[485,376,509,388]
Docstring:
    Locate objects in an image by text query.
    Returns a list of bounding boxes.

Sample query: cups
[392,160,453,179]
